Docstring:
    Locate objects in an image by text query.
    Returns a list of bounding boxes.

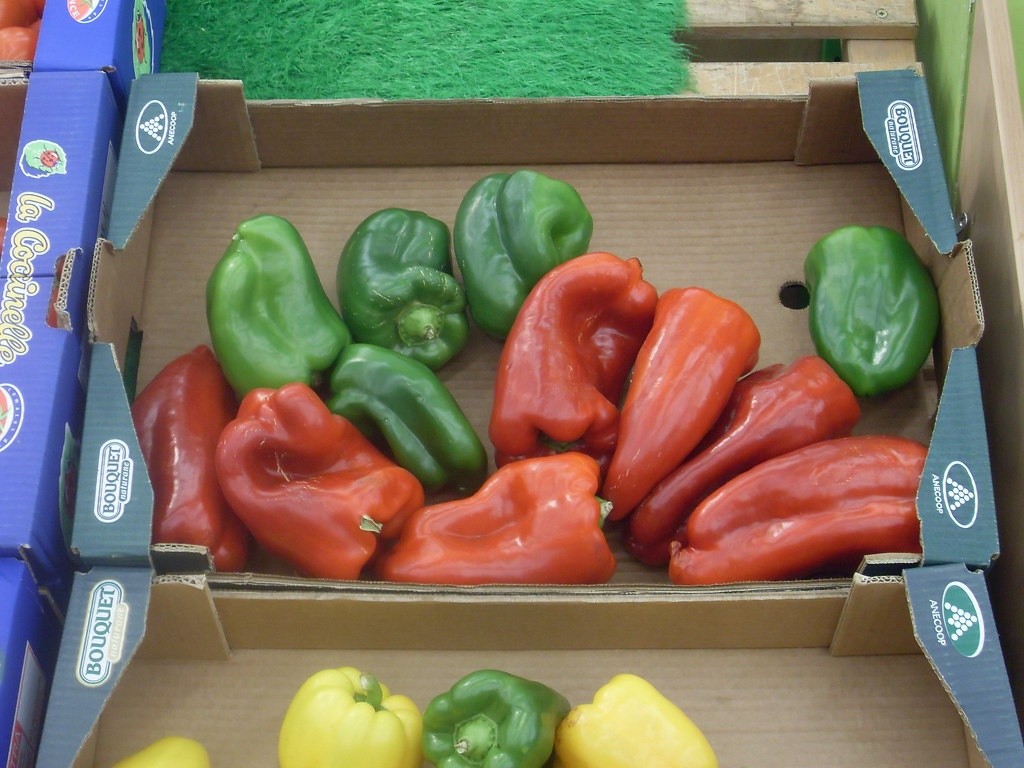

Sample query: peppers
[107,665,716,768]
[129,170,938,581]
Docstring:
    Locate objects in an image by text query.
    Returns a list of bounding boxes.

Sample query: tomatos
[0,0,45,63]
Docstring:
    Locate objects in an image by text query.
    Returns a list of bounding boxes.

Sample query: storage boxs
[0,0,1024,768]
